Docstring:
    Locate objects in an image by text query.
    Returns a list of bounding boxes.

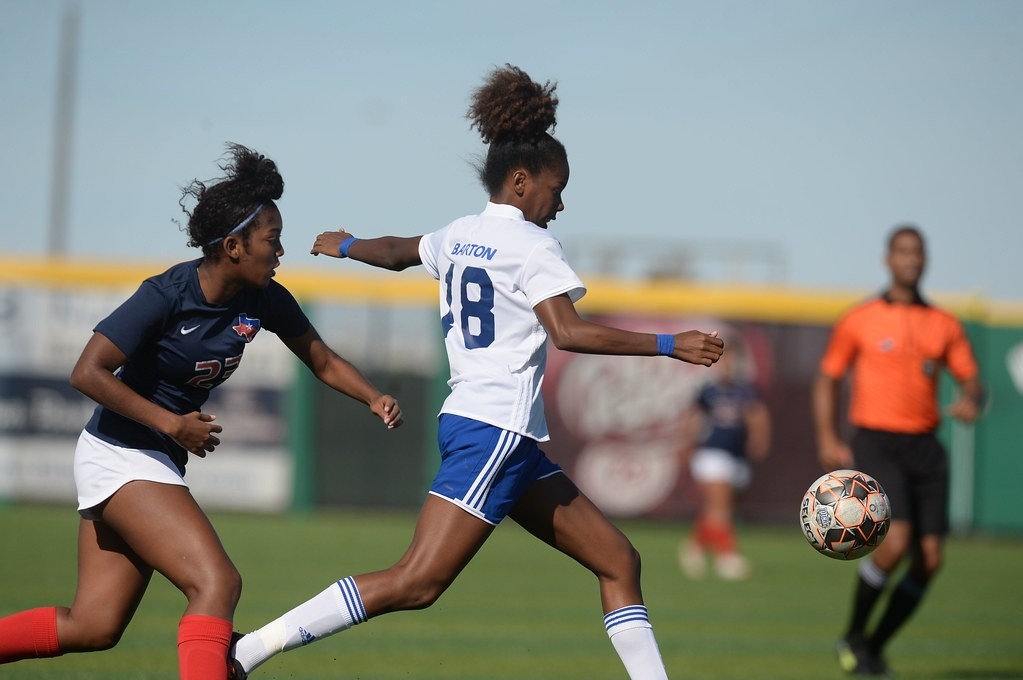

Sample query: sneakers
[227,631,247,680]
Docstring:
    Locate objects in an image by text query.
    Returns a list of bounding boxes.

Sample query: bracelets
[656,334,675,355]
[339,236,358,257]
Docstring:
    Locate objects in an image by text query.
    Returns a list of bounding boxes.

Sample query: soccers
[799,469,892,561]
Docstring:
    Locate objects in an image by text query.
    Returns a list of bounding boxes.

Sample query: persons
[812,227,987,680]
[1,142,403,680]
[676,338,772,581]
[227,66,724,680]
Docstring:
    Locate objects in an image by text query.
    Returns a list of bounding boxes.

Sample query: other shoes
[678,540,705,577]
[715,553,750,580]
[836,636,867,674]
[868,654,887,674]
